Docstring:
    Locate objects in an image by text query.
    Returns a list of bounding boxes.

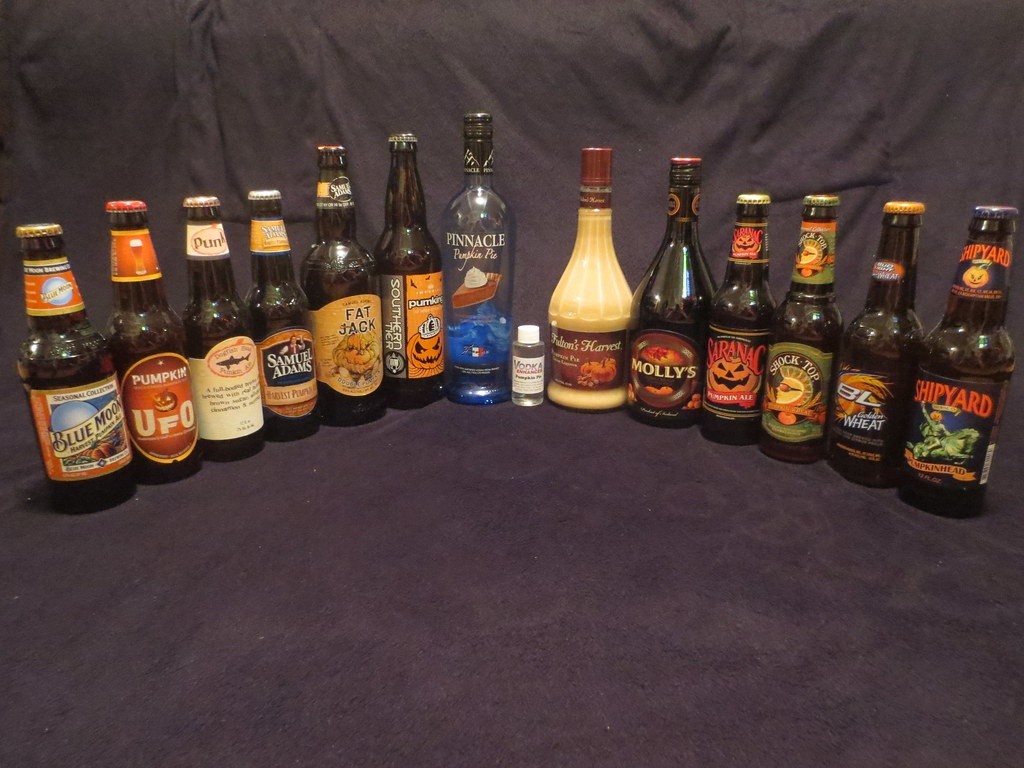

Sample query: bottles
[899,204,1021,518]
[99,200,204,484]
[626,156,721,427]
[827,200,930,489]
[754,194,843,462]
[696,192,782,446]
[173,196,268,463]
[369,134,445,407]
[436,112,518,405]
[509,324,544,407]
[12,222,137,515]
[242,190,322,442]
[546,147,634,412]
[298,145,388,427]
